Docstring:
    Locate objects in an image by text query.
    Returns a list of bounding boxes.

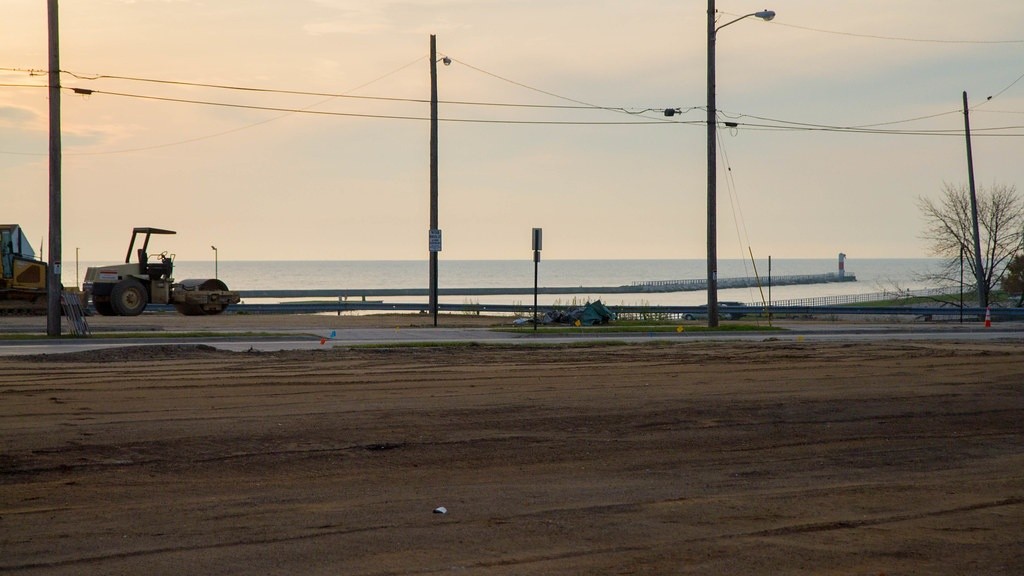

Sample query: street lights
[76,247,81,288]
[707,0,776,329]
[211,245,218,280]
[427,33,451,326]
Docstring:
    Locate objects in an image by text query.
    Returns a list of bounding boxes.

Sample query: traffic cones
[984,306,992,329]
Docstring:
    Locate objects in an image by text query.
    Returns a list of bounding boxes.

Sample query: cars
[681,299,748,322]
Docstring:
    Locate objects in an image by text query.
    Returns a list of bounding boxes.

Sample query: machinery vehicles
[0,223,94,316]
[82,227,240,316]
